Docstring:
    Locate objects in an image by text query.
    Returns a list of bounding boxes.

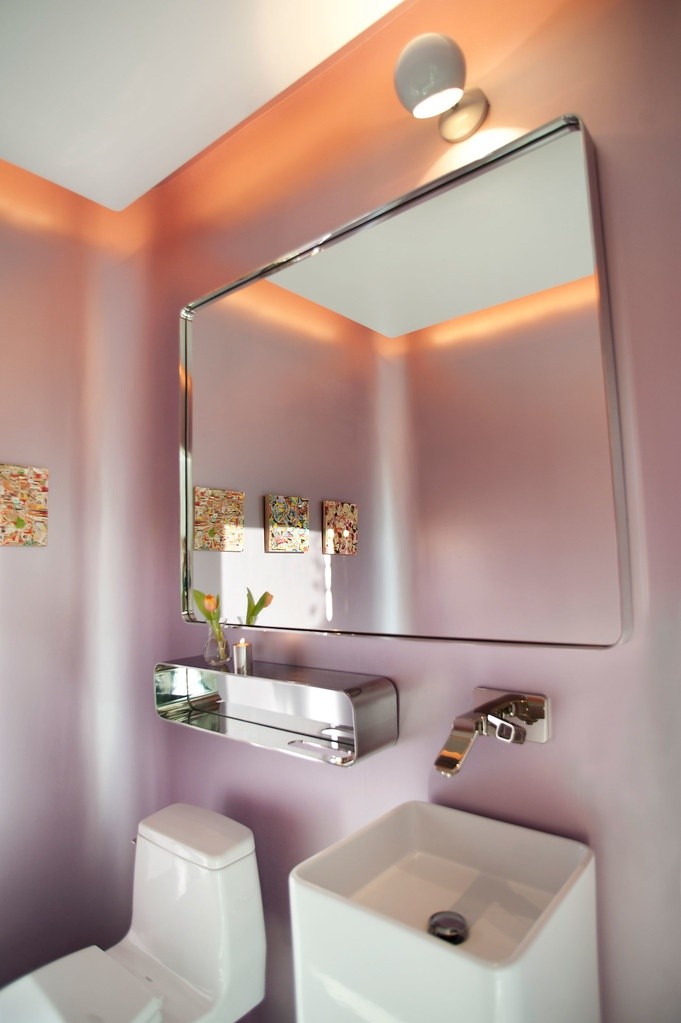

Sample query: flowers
[243,584,273,626]
[190,588,226,661]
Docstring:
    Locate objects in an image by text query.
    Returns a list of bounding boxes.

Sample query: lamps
[394,29,490,142]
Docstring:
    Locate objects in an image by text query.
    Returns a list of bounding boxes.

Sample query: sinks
[287,799,602,1022]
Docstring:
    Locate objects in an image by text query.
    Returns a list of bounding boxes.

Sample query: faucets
[433,685,553,780]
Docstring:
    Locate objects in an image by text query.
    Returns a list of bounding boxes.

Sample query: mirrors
[178,113,633,648]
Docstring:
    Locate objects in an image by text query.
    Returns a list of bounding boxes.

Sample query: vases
[236,615,256,629]
[202,616,233,668]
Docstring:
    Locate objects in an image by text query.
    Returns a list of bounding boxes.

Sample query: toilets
[0,802,269,1022]
